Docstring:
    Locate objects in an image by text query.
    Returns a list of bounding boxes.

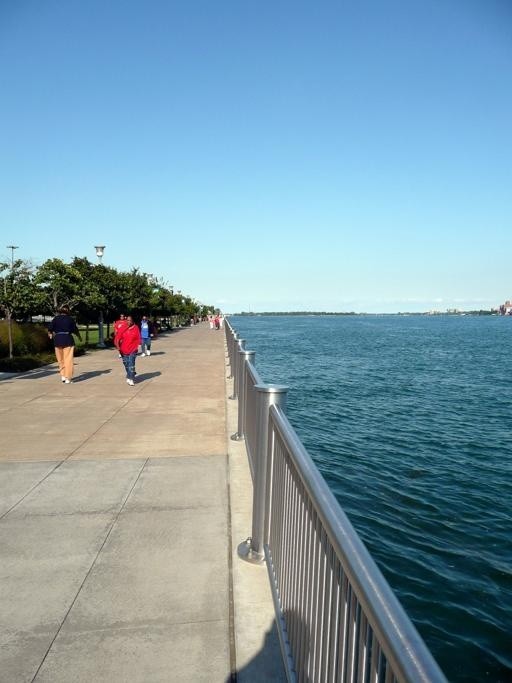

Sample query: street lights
[94,245,107,349]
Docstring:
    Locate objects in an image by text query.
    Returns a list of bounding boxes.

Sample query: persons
[138,314,155,356]
[48,303,83,384]
[209,315,214,329]
[113,311,128,357]
[214,316,220,329]
[113,316,143,386]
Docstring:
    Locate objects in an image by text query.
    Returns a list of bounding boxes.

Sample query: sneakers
[118,354,122,358]
[62,376,74,383]
[141,350,150,357]
[126,377,134,386]
